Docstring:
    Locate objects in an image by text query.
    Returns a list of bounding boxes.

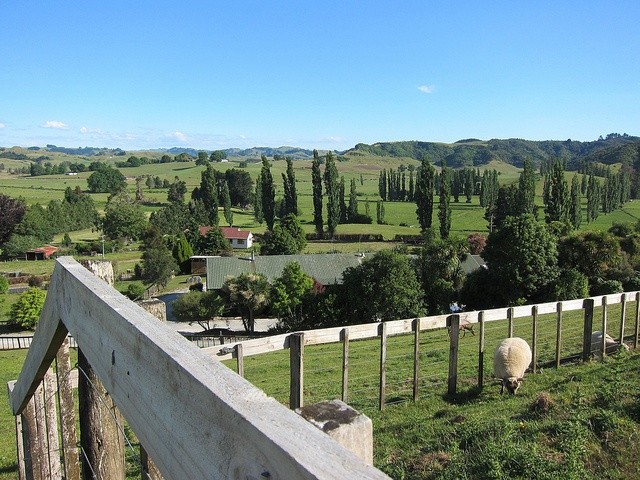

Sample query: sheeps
[493,337,532,395]
[591,331,629,352]
